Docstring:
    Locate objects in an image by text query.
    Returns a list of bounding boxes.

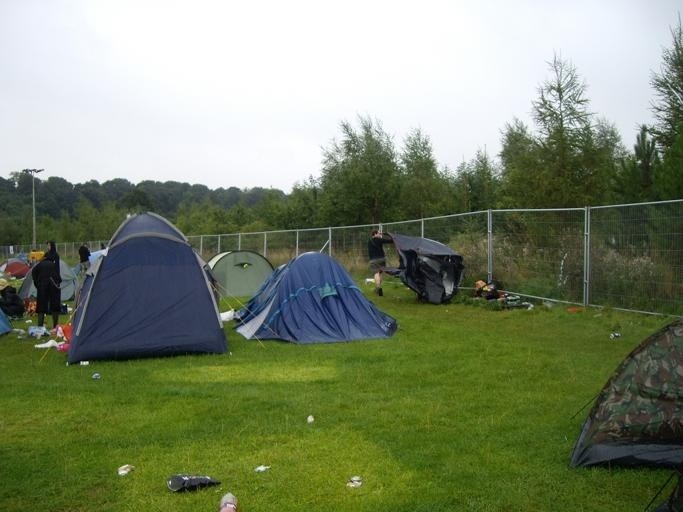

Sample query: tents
[0,251,44,277]
[383,231,465,305]
[569,318,683,471]
[233,250,398,344]
[66,209,232,364]
[70,249,106,275]
[207,249,275,298]
[17,256,77,301]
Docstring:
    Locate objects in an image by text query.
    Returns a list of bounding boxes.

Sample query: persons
[42,241,58,264]
[34,258,61,328]
[367,229,394,297]
[79,241,90,274]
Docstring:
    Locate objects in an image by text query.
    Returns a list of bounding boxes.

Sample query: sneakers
[379,288,383,296]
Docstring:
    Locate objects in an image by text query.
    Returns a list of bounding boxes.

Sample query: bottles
[218,492,237,511]
